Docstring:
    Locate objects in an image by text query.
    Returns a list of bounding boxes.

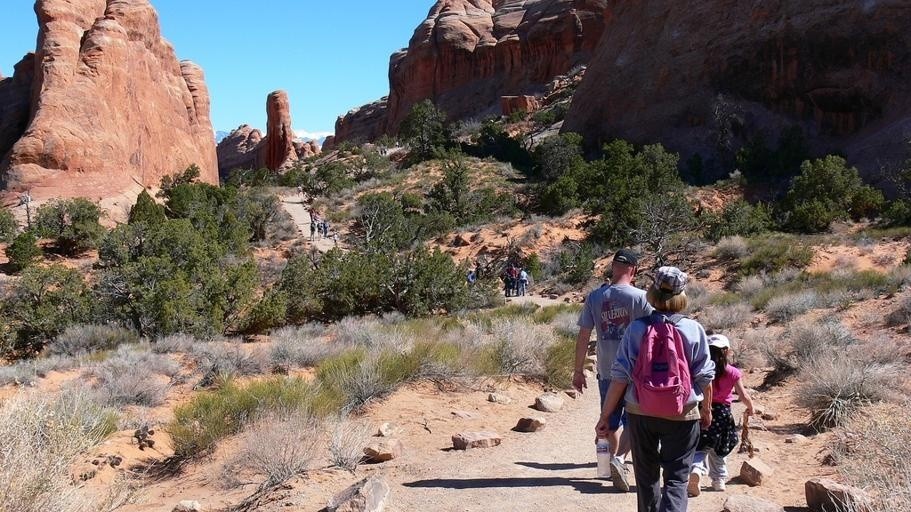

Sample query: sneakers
[711,479,726,491]
[686,467,701,497]
[609,458,631,492]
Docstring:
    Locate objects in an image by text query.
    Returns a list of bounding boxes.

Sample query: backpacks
[631,314,693,417]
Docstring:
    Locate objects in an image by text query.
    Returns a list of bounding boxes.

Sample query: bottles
[596,435,612,479]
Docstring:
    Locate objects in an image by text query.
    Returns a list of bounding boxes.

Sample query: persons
[297,185,338,243]
[376,140,399,155]
[467,266,529,297]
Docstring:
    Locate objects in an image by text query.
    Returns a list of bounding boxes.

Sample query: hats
[613,249,638,266]
[708,334,730,350]
[653,266,689,297]
